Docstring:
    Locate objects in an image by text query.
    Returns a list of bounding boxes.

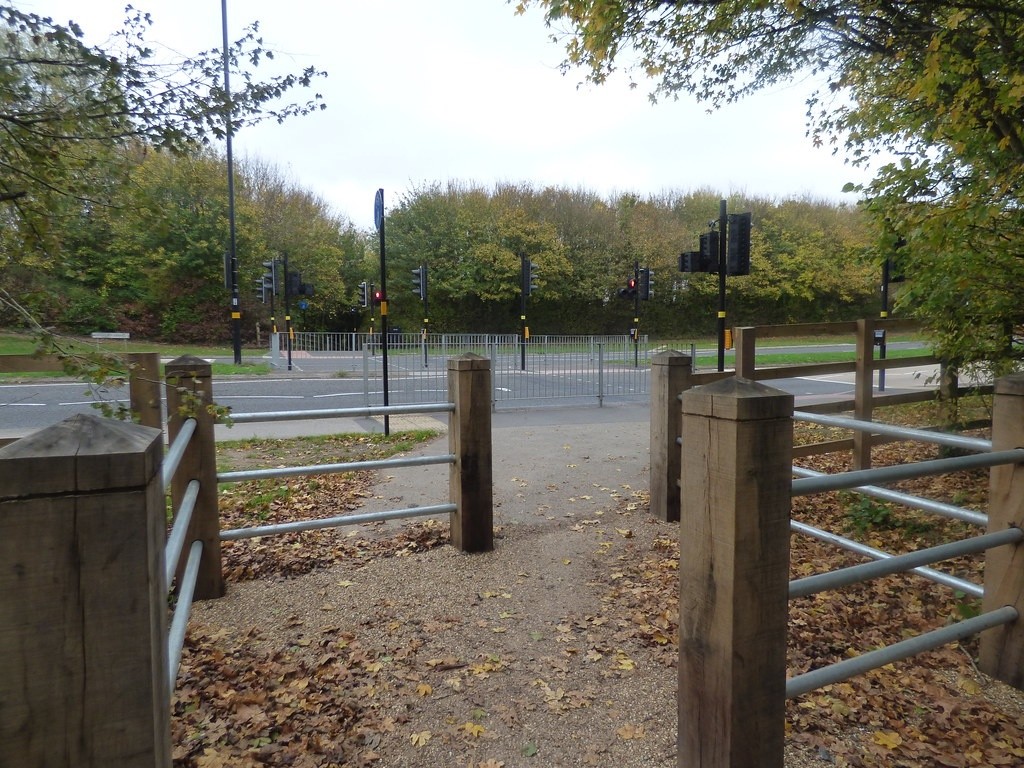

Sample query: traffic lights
[358,282,367,308]
[526,257,540,295]
[262,257,277,297]
[412,265,424,300]
[256,276,268,303]
[647,267,656,300]
[619,277,637,300]
[728,212,753,276]
[291,271,314,296]
